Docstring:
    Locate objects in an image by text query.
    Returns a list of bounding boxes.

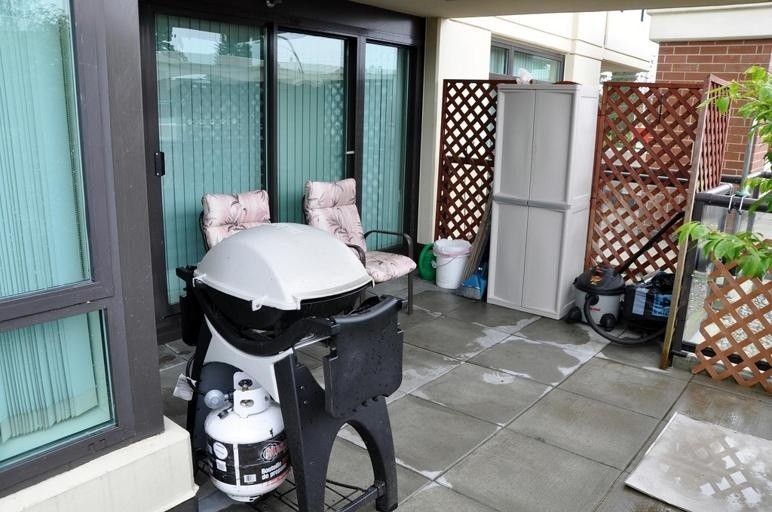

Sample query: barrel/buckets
[431,239,473,290]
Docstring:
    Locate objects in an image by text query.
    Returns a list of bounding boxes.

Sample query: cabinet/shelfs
[486,84,600,320]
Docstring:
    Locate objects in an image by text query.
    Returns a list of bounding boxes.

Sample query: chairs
[301,177,418,315]
[199,190,272,252]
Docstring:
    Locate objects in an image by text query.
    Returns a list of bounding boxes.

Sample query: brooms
[450,260,483,302]
